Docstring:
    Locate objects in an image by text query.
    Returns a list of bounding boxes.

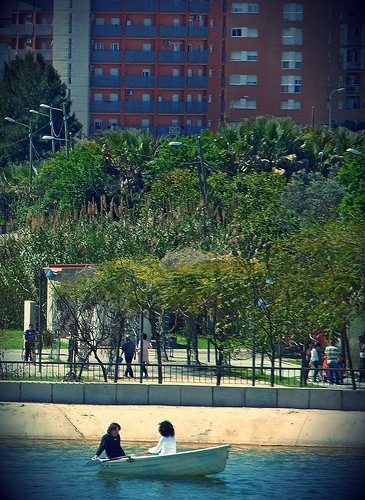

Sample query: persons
[136,420,177,456]
[355,336,365,382]
[136,332,153,379]
[120,334,136,379]
[92,422,126,460]
[304,329,345,385]
[23,324,38,366]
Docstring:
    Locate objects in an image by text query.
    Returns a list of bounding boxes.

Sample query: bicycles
[22,341,40,363]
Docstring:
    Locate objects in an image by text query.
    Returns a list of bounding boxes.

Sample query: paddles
[86,453,139,467]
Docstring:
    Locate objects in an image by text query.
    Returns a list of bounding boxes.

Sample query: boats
[98,444,231,475]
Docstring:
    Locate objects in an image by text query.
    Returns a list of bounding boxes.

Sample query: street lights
[169,135,207,200]
[38,267,55,374]
[3,102,70,190]
[328,87,345,130]
